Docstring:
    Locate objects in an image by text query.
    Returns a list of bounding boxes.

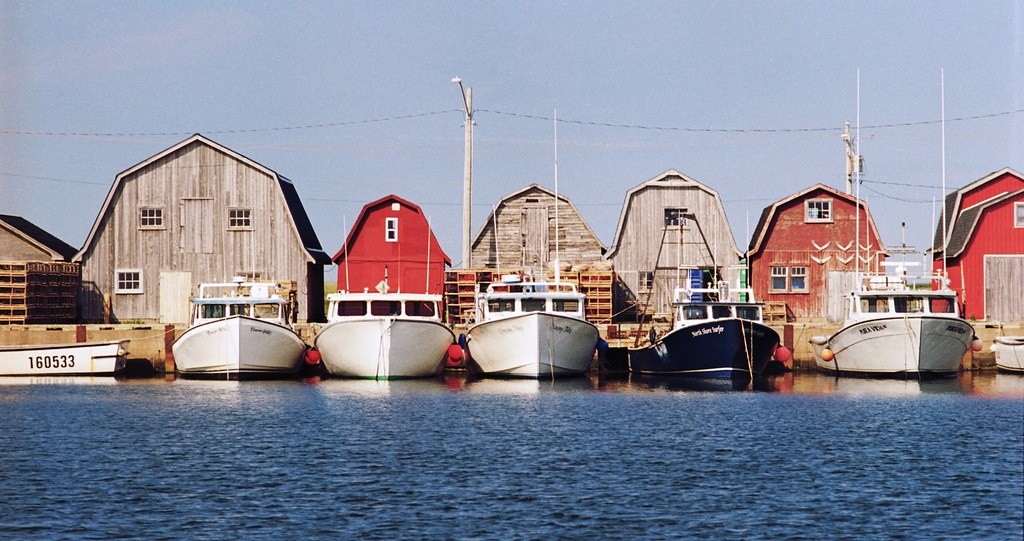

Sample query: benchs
[607,325,648,339]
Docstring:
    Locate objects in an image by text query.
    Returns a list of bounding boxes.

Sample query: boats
[992,336,1023,375]
[0,337,131,378]
[171,271,307,382]
[311,214,455,381]
[465,106,599,381]
[627,195,781,393]
[808,65,976,381]
